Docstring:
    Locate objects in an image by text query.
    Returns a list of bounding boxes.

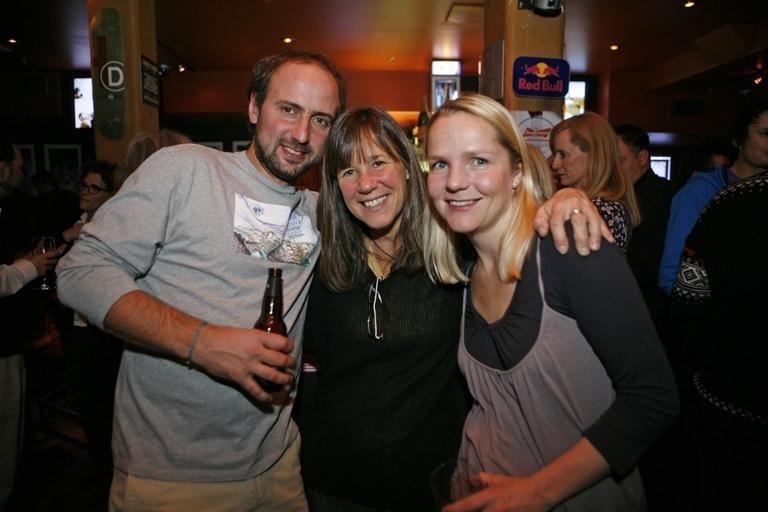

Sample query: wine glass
[428,457,485,509]
[32,236,56,290]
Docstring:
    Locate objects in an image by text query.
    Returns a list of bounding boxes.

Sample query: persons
[292,107,479,512]
[613,91,768,511]
[1,127,193,511]
[548,112,641,256]
[425,94,681,512]
[55,51,617,511]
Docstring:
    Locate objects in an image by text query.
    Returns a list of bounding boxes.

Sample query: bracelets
[187,321,207,365]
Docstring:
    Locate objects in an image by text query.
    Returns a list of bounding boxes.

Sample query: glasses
[78,181,115,196]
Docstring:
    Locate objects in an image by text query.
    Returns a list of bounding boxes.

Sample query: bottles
[251,268,287,392]
[417,96,429,147]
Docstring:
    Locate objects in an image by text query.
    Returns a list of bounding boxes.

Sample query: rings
[570,209,584,217]
